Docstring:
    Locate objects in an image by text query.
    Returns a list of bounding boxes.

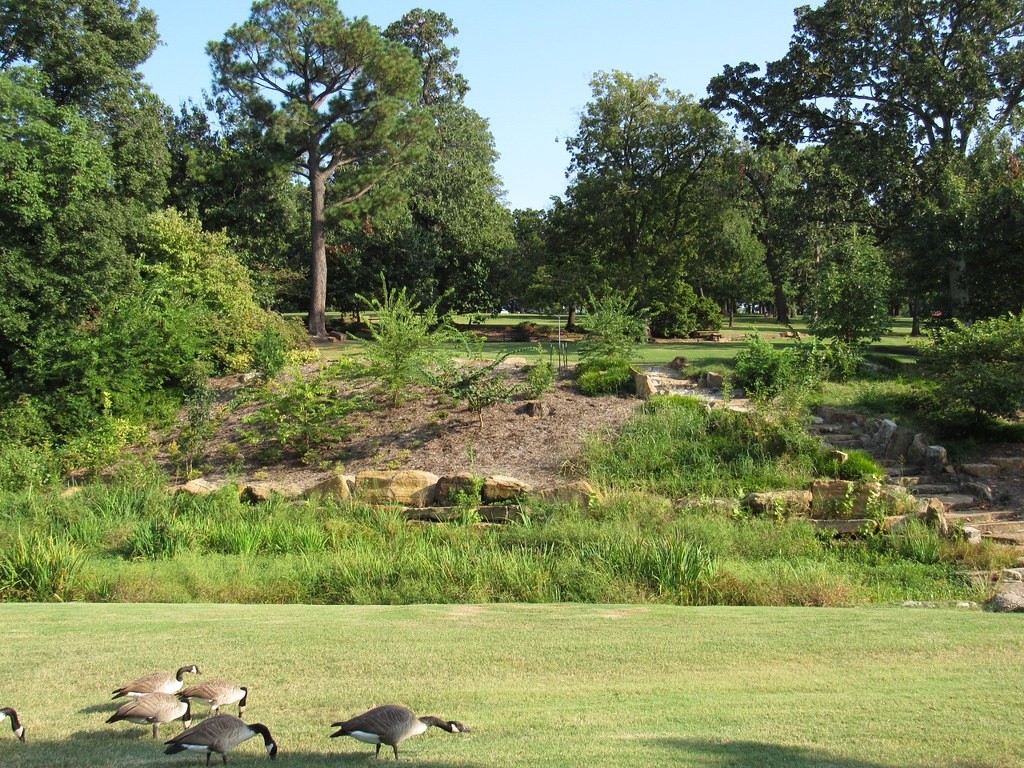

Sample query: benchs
[693,330,721,342]
[502,330,549,342]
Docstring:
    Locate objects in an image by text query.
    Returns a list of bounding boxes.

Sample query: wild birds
[113,661,469,768]
[0,706,27,745]
